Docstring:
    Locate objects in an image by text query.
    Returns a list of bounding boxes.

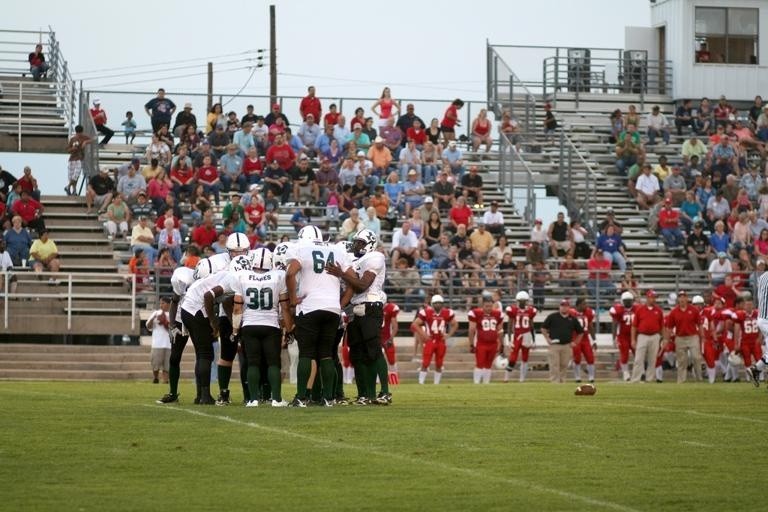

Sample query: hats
[756,259,765,267]
[408,169,415,175]
[718,251,727,258]
[93,98,101,105]
[665,198,672,203]
[357,151,364,156]
[607,209,614,216]
[353,122,361,129]
[646,289,658,297]
[677,290,687,296]
[491,200,498,206]
[694,221,703,227]
[248,183,261,191]
[184,103,191,109]
[424,195,432,203]
[560,299,569,305]
[535,218,543,224]
[375,135,383,143]
[272,104,280,110]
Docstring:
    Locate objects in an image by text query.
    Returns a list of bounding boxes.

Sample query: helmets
[297,225,322,243]
[352,228,376,257]
[225,231,250,250]
[251,247,272,271]
[228,255,251,272]
[273,242,295,270]
[620,292,633,312]
[192,257,219,279]
[430,294,444,313]
[692,295,704,304]
[516,291,529,309]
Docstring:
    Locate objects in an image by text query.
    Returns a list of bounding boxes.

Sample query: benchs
[510,83,712,266]
[203,180,397,253]
[0,66,148,321]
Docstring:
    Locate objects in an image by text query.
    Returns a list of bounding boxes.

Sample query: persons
[65,85,768,408]
[0,164,61,300]
[716,53,725,63]
[27,42,52,81]
[695,42,711,63]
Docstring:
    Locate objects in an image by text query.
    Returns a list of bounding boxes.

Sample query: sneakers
[156,393,180,404]
[316,396,334,407]
[246,399,259,407]
[215,398,232,406]
[265,397,272,403]
[349,396,369,406]
[271,397,289,407]
[288,397,307,408]
[336,397,348,406]
[199,397,216,405]
[746,364,761,387]
[372,392,392,405]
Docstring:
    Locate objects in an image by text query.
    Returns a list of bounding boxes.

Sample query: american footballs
[574,383,597,396]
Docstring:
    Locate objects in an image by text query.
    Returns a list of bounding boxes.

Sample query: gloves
[229,332,241,343]
[169,326,182,344]
[284,331,294,345]
